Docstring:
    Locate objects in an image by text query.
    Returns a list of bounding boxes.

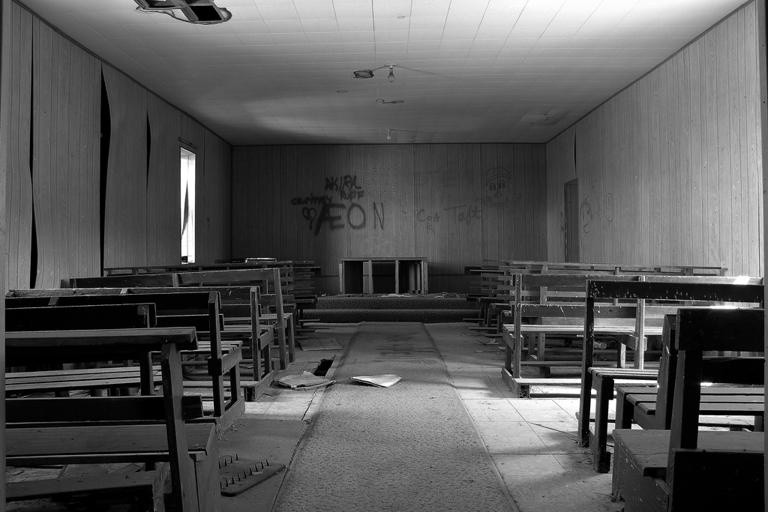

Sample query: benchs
[7,267,295,417]
[0,327,216,506]
[167,259,320,332]
[481,259,763,399]
[575,279,768,512]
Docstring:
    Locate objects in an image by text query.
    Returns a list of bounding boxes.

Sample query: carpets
[268,320,519,508]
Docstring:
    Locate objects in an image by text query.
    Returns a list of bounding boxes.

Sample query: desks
[338,257,430,296]
